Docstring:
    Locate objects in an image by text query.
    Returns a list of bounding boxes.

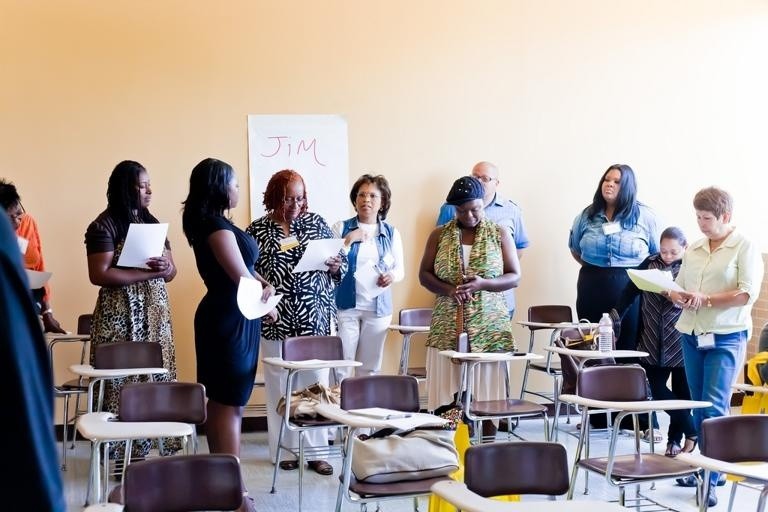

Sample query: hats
[446,175,486,206]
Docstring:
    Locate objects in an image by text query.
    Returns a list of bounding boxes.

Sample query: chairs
[261,306,767,510]
[47,330,255,510]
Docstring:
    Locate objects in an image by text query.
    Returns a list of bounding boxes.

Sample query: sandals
[308,460,333,475]
[666,441,680,458]
[644,428,663,443]
[282,460,298,470]
[683,435,698,453]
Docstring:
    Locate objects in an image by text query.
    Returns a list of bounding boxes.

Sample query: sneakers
[697,471,718,506]
[676,474,727,487]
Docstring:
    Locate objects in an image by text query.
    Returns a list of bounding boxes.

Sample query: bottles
[597,313,614,351]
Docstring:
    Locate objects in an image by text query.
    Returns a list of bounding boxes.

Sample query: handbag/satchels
[274,383,342,421]
[351,425,460,485]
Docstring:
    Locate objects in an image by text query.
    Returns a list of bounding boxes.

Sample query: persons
[0,175,69,336]
[416,176,524,444]
[179,157,282,512]
[325,174,407,445]
[567,162,665,445]
[664,178,765,508]
[0,205,69,511]
[594,225,699,460]
[81,156,185,480]
[244,168,347,477]
[436,161,530,433]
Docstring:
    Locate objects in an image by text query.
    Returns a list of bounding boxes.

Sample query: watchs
[40,307,54,318]
[706,294,712,308]
